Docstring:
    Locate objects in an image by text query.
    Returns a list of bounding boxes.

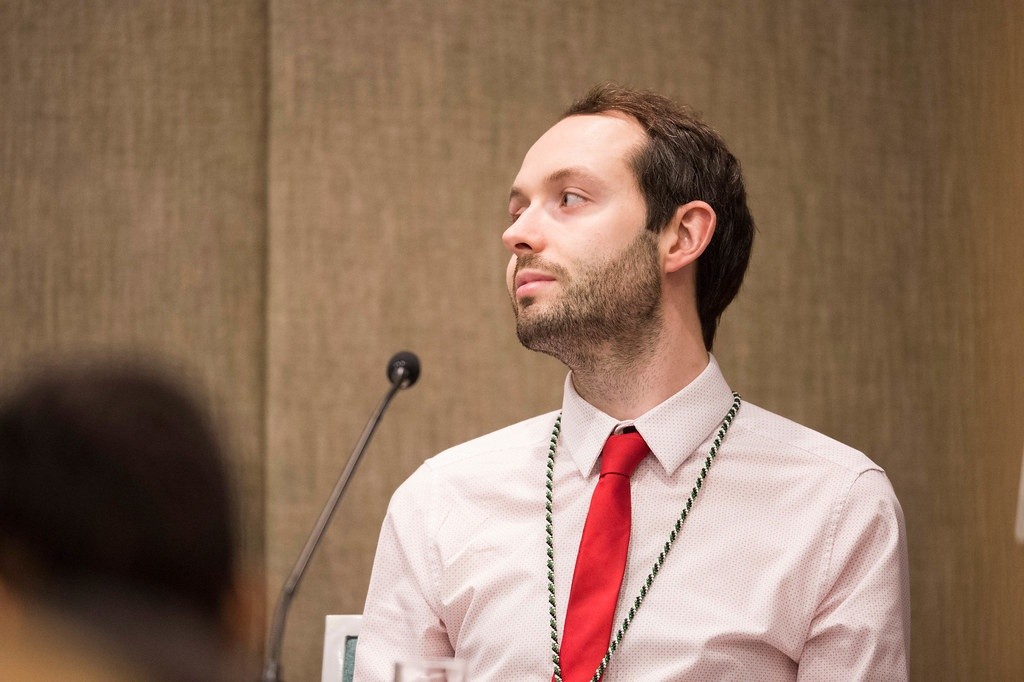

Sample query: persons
[353,83,912,682]
[0,336,256,682]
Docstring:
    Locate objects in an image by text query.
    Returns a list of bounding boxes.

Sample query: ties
[551,429,650,682]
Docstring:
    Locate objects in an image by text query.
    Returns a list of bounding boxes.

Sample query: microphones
[264,352,421,682]
